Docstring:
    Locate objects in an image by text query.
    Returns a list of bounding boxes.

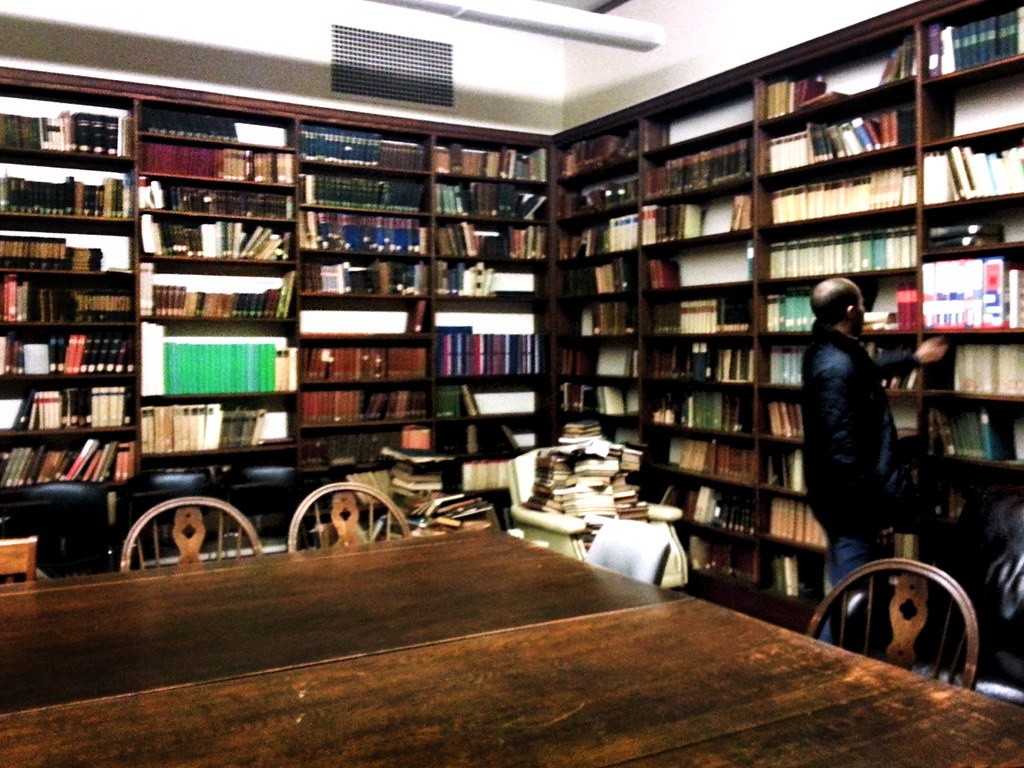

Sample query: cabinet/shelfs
[0,0,1024,705]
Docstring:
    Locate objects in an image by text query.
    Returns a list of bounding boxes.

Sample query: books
[536,3,1024,261]
[0,331,551,433]
[0,422,692,595]
[555,256,1024,463]
[0,104,552,226]
[1,226,548,333]
[608,429,965,625]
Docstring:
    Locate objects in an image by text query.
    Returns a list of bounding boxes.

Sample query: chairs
[1,463,411,581]
[804,557,984,689]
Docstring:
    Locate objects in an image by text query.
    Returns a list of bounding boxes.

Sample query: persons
[799,277,946,645]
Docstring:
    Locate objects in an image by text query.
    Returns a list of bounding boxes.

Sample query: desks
[0,530,1023,768]
